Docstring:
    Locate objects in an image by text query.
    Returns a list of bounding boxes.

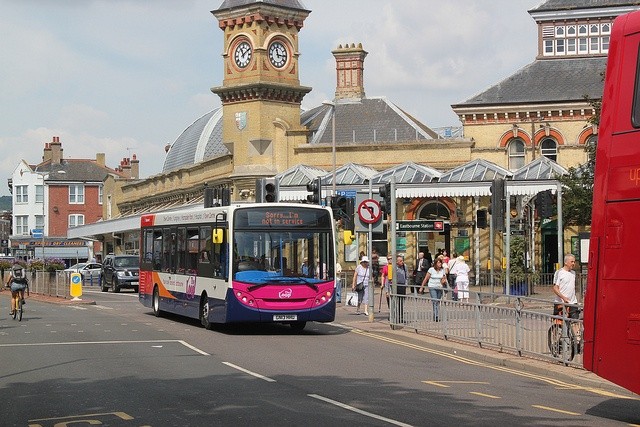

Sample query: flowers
[0,258,9,269]
[44,256,67,272]
[9,258,28,269]
[28,258,44,272]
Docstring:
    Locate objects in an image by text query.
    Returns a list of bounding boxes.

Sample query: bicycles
[546,303,584,361]
[5,278,30,320]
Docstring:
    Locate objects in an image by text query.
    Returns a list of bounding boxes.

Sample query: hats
[456,255,465,264]
[360,256,370,264]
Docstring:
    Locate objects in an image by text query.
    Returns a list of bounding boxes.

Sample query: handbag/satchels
[355,282,365,293]
[345,291,358,307]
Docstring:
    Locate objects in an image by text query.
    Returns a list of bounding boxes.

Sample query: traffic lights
[307,182,317,203]
[377,183,391,214]
[486,178,505,230]
[334,196,346,220]
[264,182,276,201]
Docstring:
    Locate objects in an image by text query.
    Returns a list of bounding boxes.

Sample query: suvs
[99,252,140,292]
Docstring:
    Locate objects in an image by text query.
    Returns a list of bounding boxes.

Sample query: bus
[138,202,338,330]
[578,11,639,396]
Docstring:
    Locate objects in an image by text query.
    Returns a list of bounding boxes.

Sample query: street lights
[18,168,67,258]
[511,113,551,161]
[321,99,336,201]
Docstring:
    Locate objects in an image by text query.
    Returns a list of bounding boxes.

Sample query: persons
[301,257,309,276]
[372,246,379,287]
[382,257,392,308]
[438,255,449,296]
[357,251,365,265]
[438,248,451,292]
[5,261,29,314]
[448,253,459,300]
[454,256,471,306]
[435,249,442,258]
[396,255,409,324]
[554,254,579,321]
[378,248,388,273]
[414,252,429,294]
[418,257,447,322]
[352,256,373,315]
[91,255,96,263]
[316,259,327,280]
[336,259,342,303]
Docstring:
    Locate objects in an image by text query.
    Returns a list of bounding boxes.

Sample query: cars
[64,261,104,279]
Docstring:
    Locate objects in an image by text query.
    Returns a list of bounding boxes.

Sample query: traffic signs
[396,220,444,232]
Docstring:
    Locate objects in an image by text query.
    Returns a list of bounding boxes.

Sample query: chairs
[274,257,287,269]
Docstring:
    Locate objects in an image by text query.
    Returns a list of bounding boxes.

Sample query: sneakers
[21,298,25,304]
[363,311,369,316]
[9,310,14,315]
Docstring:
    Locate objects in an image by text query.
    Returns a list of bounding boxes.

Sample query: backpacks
[9,264,29,296]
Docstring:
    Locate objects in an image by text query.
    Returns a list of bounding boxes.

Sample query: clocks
[234,42,252,68]
[269,42,287,68]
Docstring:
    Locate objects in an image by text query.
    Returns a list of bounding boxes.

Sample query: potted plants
[501,235,540,296]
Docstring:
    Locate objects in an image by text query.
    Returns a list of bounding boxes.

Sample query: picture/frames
[571,236,580,261]
